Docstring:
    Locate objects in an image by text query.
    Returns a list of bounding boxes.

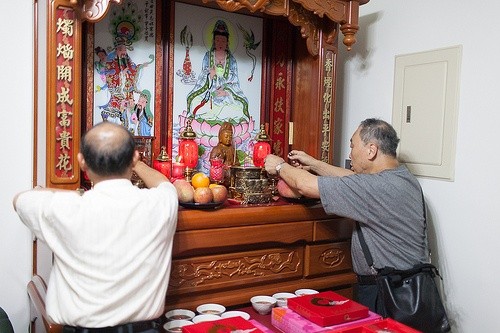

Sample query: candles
[288,122,294,144]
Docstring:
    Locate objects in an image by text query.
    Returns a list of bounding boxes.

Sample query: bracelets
[275,161,288,175]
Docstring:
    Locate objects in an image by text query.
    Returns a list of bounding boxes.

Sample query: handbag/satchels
[378,262,450,332]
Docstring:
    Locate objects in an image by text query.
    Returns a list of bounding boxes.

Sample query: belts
[62,320,157,333]
[356,275,378,285]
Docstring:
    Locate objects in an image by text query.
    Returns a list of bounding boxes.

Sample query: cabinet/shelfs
[165,199,356,316]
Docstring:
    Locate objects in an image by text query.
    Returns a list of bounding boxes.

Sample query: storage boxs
[181,291,422,333]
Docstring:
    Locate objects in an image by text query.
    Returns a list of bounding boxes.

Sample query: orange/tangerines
[192,172,210,188]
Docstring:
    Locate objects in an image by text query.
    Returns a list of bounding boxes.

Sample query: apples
[172,180,227,204]
[278,179,304,198]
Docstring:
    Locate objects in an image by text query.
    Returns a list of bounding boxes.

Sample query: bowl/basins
[272,292,298,306]
[251,295,278,314]
[295,289,319,296]
[163,320,194,333]
[192,314,221,323]
[165,308,195,320]
[196,303,226,316]
[221,310,250,320]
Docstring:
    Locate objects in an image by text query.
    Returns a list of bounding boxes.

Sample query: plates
[181,201,223,209]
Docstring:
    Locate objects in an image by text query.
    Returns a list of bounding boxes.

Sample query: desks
[158,305,281,333]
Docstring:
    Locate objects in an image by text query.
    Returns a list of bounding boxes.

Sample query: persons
[13,121,179,333]
[264,119,432,320]
[209,122,240,188]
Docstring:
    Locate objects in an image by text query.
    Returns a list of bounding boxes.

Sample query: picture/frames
[83,0,272,178]
